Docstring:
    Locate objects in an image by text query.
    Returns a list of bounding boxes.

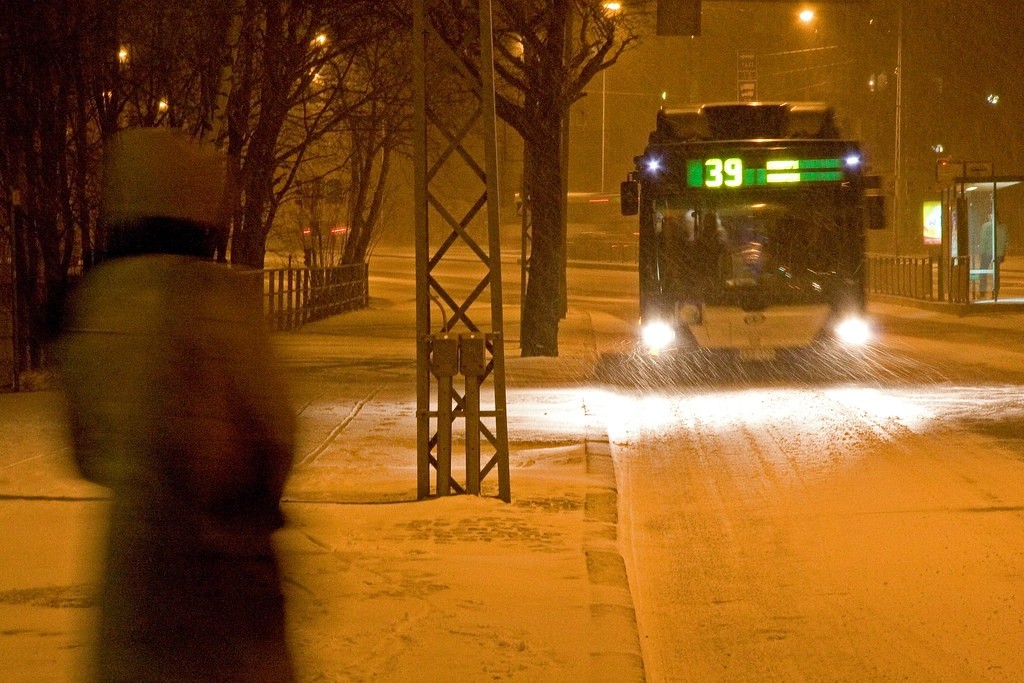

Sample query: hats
[105,127,224,224]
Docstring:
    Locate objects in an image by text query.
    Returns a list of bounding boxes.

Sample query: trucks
[622,96,884,353]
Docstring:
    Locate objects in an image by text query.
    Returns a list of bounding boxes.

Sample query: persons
[57,128,304,683]
[975,208,1007,301]
